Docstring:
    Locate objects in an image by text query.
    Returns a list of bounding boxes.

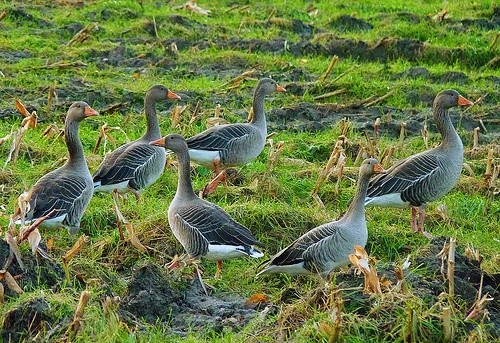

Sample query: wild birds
[256,158,393,286]
[347,90,476,241]
[91,84,183,209]
[149,133,268,281]
[165,78,286,199]
[14,101,99,249]
